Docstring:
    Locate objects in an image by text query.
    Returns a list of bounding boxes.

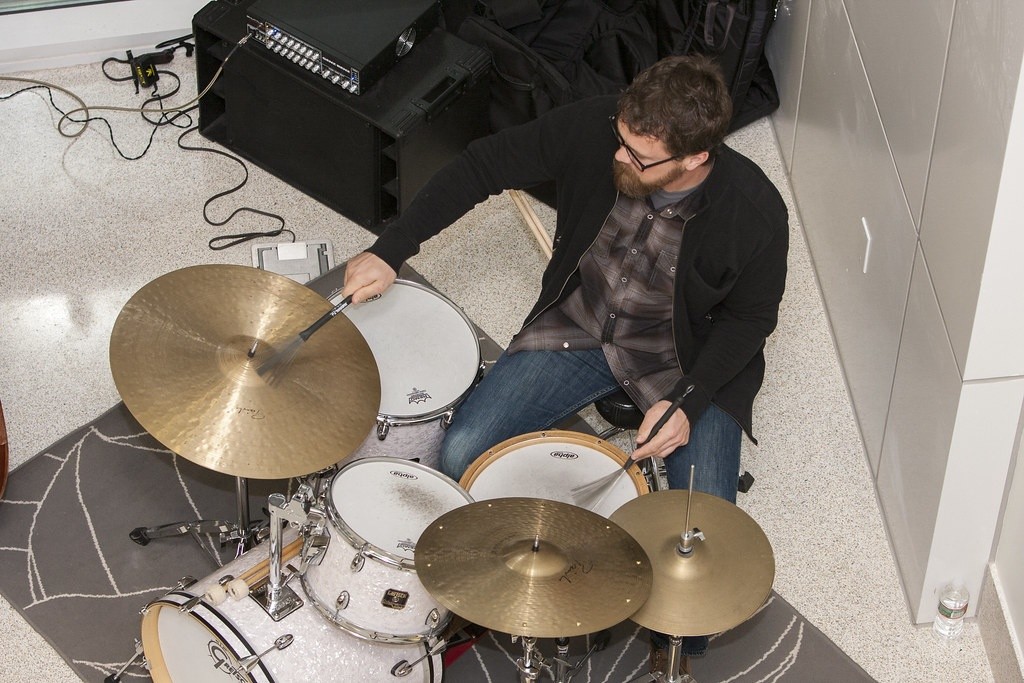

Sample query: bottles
[933,579,970,641]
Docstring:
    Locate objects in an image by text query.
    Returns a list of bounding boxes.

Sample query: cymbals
[109,264,382,480]
[414,496,654,638]
[606,489,776,638]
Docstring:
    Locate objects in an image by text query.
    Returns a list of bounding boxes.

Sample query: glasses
[608,110,678,172]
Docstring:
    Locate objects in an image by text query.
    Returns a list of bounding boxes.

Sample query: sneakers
[440,615,470,641]
[650,648,691,678]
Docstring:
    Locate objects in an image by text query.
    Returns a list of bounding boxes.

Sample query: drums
[297,455,478,647]
[456,430,651,522]
[294,278,487,498]
[133,521,444,683]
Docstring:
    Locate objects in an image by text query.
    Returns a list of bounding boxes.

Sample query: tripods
[130,478,264,564]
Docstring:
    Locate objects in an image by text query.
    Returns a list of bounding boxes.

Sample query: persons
[342,49,789,504]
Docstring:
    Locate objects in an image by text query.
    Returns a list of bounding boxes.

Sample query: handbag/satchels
[439,0,781,136]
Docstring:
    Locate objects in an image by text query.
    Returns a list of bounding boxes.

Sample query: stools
[592,387,755,509]
